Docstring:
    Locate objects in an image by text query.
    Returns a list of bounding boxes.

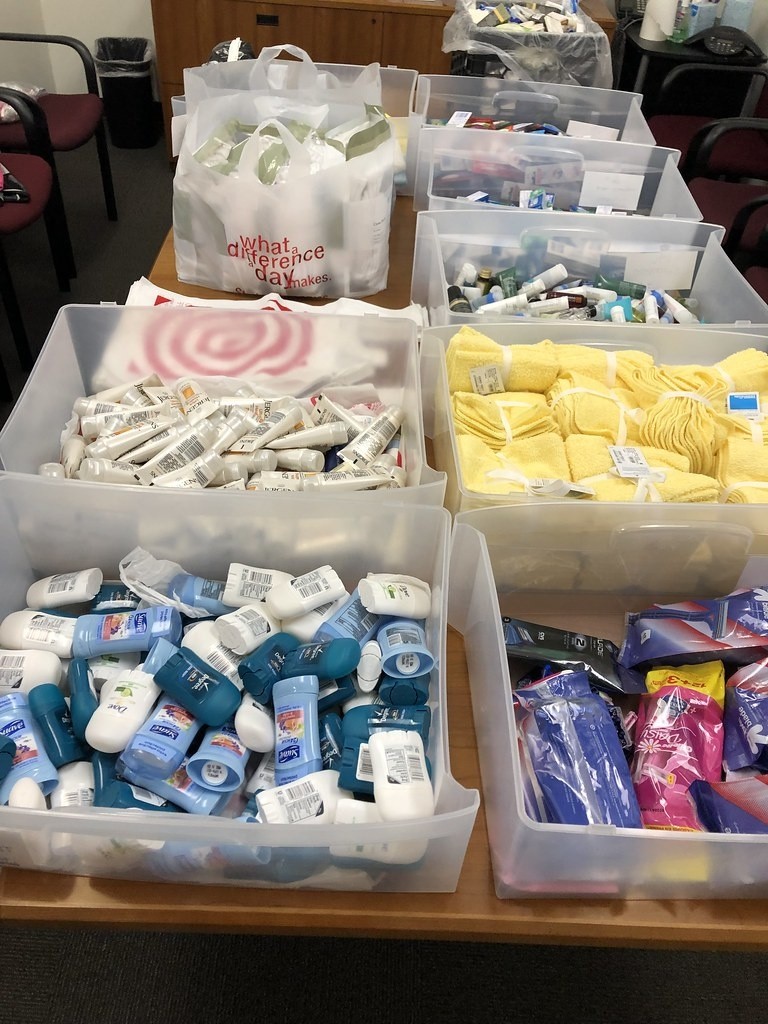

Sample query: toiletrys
[1,560,435,883]
[442,261,701,326]
[15,375,406,576]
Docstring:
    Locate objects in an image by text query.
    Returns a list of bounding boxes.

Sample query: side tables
[618,18,768,121]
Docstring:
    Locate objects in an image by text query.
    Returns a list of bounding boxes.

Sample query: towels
[433,322,767,587]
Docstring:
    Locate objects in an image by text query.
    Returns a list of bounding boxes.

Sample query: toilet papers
[638,0,679,41]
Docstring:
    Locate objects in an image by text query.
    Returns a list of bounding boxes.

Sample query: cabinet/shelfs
[151,0,616,172]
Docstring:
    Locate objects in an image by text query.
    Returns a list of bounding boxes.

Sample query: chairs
[1,90,70,374]
[0,32,118,280]
[640,62,767,305]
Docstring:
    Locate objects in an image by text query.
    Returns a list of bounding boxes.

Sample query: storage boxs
[0,303,447,506]
[446,502,768,900]
[273,57,423,195]
[409,209,768,337]
[420,323,767,533]
[1,474,481,894]
[413,126,704,221]
[414,73,657,145]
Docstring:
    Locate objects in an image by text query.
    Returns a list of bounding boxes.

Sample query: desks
[0,192,768,948]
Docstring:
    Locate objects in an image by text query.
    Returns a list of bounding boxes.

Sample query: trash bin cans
[91,35,166,148]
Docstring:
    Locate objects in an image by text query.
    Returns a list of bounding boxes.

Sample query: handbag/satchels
[170,43,397,301]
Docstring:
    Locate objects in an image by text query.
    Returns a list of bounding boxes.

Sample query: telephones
[615,0,647,19]
